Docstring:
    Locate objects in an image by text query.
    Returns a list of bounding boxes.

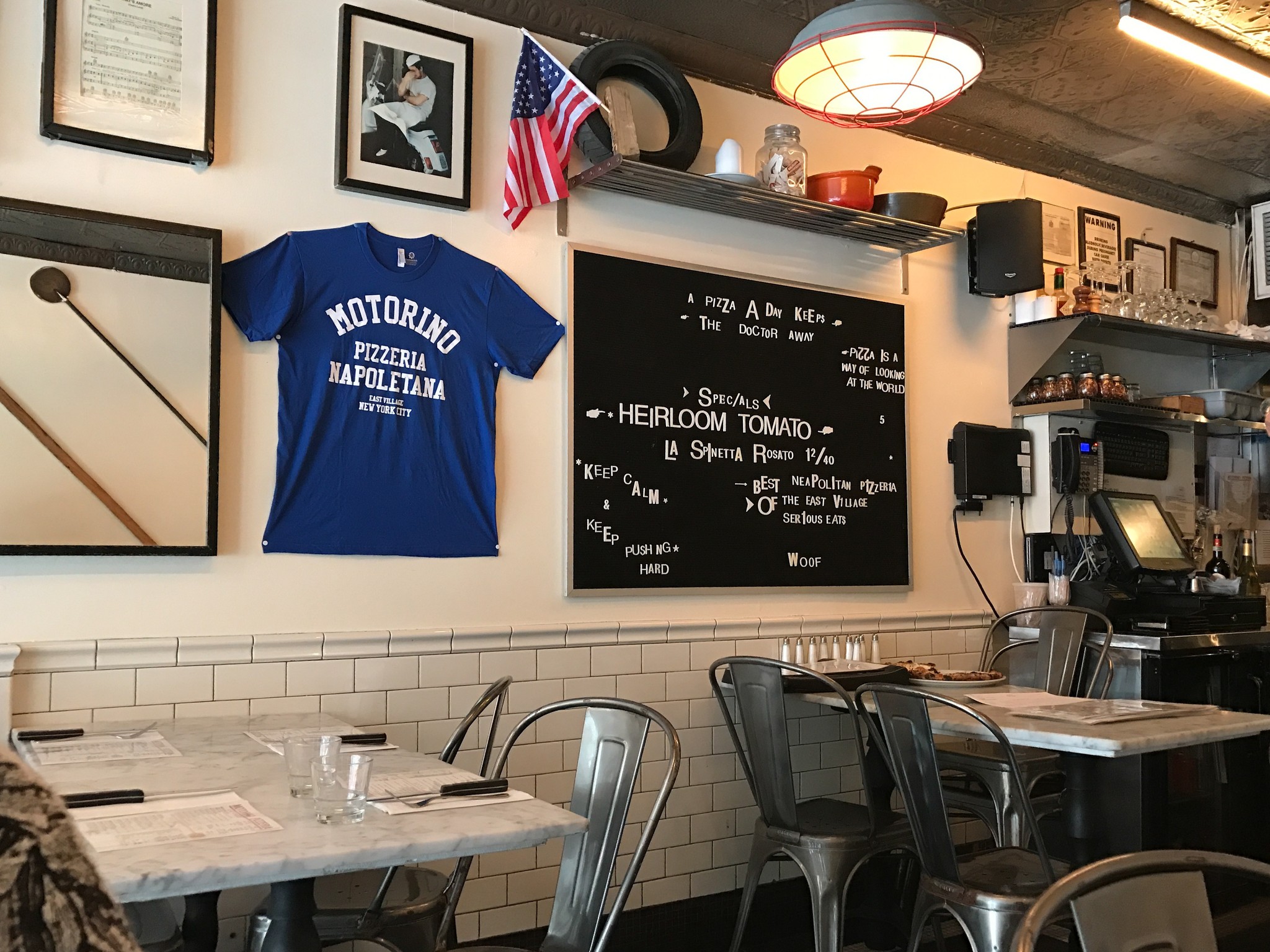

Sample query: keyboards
[1092,421,1170,480]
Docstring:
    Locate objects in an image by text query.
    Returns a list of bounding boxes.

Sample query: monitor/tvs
[1090,489,1197,577]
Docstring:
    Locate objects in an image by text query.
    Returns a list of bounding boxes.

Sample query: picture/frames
[1168,235,1220,310]
[334,2,473,211]
[2,196,227,562]
[563,239,914,591]
[1076,206,1124,294]
[1125,235,1168,299]
[39,1,220,166]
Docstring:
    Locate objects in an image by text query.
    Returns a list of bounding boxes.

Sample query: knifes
[59,785,240,809]
[262,733,387,745]
[366,779,509,802]
[17,727,158,741]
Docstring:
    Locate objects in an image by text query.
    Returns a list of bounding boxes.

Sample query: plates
[909,670,1006,687]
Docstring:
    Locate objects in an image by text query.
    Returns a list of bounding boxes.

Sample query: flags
[503,34,599,230]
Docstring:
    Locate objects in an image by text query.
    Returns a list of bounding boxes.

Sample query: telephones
[1051,427,1103,496]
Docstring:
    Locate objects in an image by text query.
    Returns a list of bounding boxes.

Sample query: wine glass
[1061,260,1210,330]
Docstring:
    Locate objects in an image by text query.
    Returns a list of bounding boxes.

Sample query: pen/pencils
[1050,545,1066,577]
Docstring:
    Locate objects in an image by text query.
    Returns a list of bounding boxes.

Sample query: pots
[805,165,948,244]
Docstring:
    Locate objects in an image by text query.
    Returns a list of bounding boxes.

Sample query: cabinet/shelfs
[1013,310,1270,434]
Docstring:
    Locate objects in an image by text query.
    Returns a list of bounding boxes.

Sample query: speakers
[967,199,1045,297]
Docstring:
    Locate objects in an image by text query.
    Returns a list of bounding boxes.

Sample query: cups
[283,734,342,799]
[1047,571,1070,606]
[310,753,374,825]
[1013,582,1049,629]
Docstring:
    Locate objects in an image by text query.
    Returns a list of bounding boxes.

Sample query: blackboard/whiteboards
[564,239,915,597]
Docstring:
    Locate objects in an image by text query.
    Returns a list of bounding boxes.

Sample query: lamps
[765,1,991,131]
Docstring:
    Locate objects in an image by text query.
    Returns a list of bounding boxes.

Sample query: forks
[35,721,158,743]
[385,788,509,808]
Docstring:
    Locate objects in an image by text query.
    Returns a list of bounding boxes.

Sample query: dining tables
[20,701,594,950]
[717,666,1268,950]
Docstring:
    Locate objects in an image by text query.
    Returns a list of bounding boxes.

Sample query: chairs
[244,677,522,949]
[853,678,1100,952]
[707,654,988,952]
[938,599,1116,866]
[999,846,1270,946]
[338,688,691,952]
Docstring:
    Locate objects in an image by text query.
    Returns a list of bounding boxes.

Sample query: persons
[366,54,437,156]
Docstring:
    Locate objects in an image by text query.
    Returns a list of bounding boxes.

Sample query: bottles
[1037,267,1068,317]
[782,633,880,664]
[1205,534,1261,596]
